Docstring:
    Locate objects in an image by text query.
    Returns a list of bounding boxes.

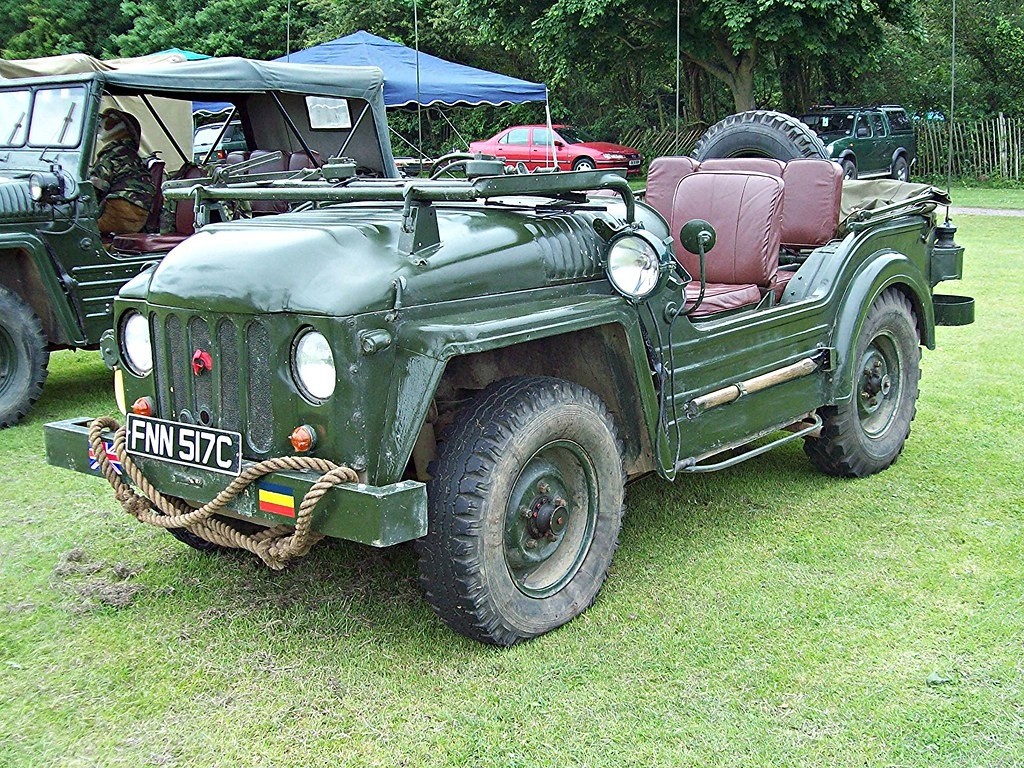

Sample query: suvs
[794,105,919,182]
[1,47,405,434]
[40,111,976,649]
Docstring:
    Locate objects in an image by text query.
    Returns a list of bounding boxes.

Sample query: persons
[88,107,154,233]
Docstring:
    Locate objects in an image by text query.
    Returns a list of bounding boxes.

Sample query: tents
[191,29,561,171]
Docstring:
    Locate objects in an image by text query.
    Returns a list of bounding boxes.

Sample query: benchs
[646,155,843,271]
[221,149,321,222]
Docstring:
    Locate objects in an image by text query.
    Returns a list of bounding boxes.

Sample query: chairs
[111,164,208,254]
[101,156,165,253]
[669,171,787,318]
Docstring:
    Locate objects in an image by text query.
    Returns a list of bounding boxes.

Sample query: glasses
[97,112,120,130]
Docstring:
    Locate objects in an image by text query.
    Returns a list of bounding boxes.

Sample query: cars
[468,123,645,182]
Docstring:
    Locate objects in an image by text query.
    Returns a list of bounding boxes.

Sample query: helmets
[103,108,141,152]
